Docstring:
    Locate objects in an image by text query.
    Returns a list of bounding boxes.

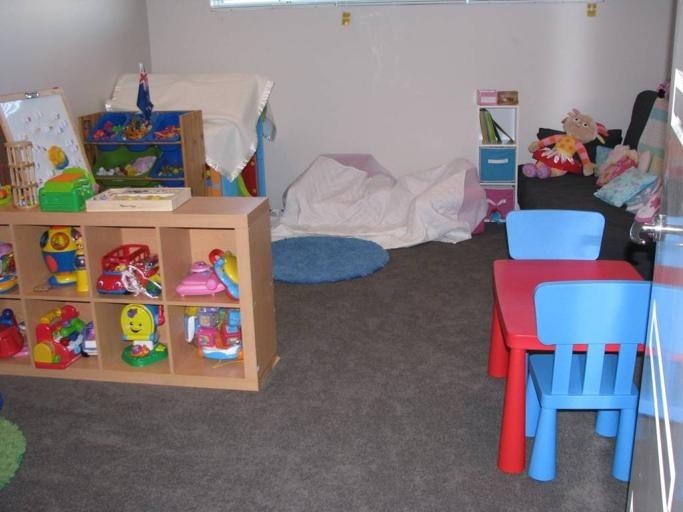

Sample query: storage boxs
[476,90,497,105]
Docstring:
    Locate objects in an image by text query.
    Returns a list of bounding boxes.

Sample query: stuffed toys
[523,107,607,178]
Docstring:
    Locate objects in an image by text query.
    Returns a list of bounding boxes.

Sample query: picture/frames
[0,86,96,184]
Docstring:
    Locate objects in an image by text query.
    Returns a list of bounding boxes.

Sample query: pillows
[595,146,613,178]
[592,166,658,208]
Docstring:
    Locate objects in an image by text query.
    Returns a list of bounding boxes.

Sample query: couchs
[518,90,669,267]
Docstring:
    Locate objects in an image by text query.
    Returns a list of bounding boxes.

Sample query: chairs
[505,210,604,385]
[525,280,650,481]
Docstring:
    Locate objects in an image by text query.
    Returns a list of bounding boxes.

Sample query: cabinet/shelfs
[0,197,280,392]
[78,110,207,197]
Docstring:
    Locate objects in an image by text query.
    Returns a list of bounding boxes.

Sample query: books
[479,110,499,144]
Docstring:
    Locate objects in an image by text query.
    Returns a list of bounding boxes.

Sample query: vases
[658,90,664,98]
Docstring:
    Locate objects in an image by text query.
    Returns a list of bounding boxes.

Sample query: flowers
[655,80,670,96]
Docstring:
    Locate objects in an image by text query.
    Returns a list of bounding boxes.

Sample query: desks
[488,260,644,473]
